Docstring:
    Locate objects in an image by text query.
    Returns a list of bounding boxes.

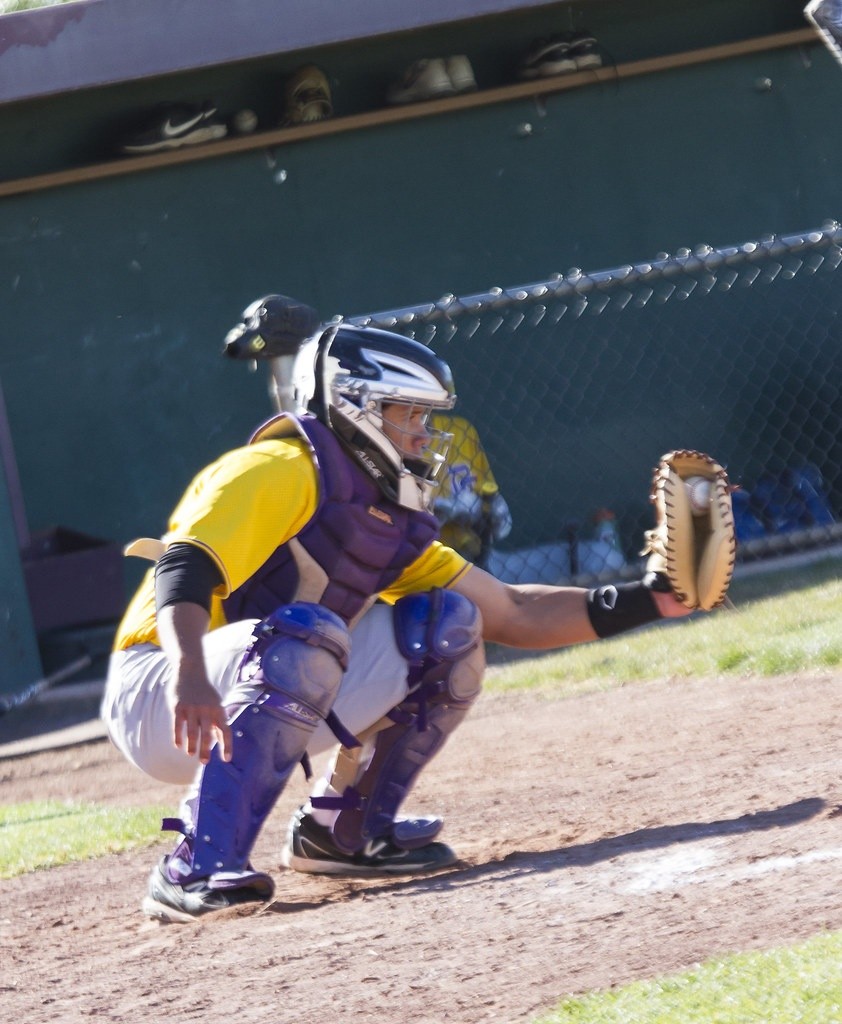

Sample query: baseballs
[685,473,714,520]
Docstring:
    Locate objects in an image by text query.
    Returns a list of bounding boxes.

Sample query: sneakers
[109,100,229,157]
[141,854,276,922]
[514,26,601,80]
[283,807,458,876]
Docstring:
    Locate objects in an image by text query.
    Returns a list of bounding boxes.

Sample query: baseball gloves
[648,448,736,612]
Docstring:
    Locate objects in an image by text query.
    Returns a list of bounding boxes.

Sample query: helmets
[291,322,458,514]
[222,293,320,359]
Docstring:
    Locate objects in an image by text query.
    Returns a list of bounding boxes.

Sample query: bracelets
[585,581,663,638]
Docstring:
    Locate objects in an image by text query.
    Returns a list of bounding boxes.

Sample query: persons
[425,416,513,578]
[101,323,697,918]
[729,463,833,544]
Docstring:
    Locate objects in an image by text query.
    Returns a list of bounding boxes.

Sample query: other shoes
[383,52,479,107]
[271,59,336,129]
